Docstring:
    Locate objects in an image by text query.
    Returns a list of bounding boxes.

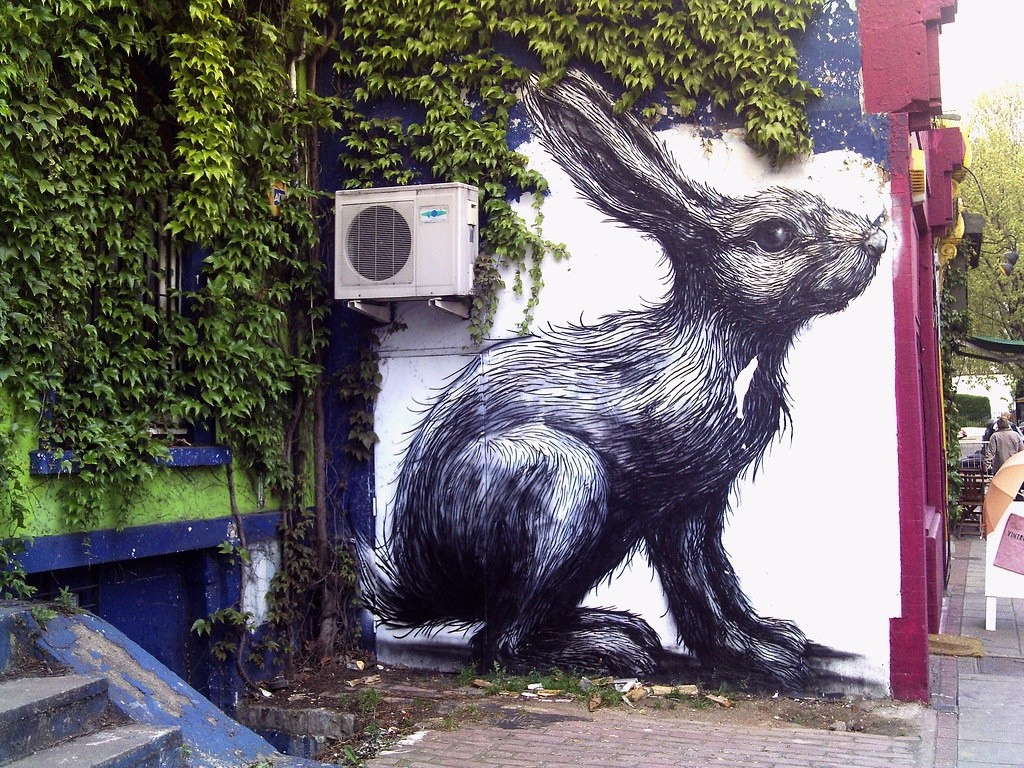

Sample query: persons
[980,410,1024,478]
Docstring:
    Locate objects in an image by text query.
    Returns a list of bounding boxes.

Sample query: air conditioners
[336,182,479,303]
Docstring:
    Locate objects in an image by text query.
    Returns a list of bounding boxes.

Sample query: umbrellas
[983,449,1024,536]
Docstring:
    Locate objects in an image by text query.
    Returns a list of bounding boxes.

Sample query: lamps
[981,247,1014,278]
[984,233,1020,265]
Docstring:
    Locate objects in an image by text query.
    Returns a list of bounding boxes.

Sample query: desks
[960,475,996,531]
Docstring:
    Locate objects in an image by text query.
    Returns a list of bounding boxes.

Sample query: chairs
[958,468,986,539]
